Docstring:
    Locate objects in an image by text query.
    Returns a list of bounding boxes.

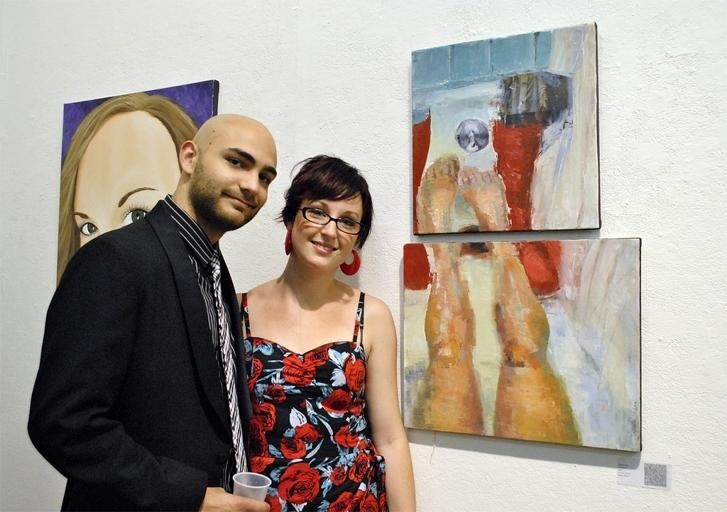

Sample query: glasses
[298,206,365,235]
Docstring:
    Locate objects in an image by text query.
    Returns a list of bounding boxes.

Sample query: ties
[210,249,249,473]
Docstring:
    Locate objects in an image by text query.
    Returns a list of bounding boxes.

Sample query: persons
[221,152,420,511]
[410,151,583,448]
[53,93,199,266]
[25,113,282,510]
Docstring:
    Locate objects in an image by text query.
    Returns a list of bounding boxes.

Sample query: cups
[233,472,272,501]
